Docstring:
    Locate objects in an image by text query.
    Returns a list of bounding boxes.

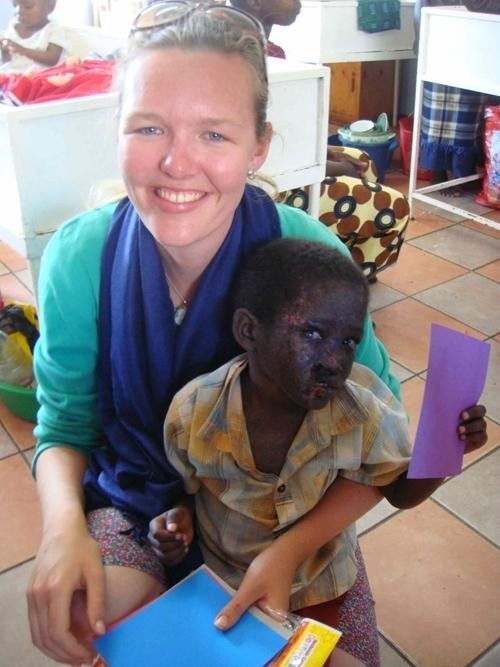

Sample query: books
[90,564,342,666]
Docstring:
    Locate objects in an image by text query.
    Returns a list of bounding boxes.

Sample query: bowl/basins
[349,120,375,133]
[337,127,397,145]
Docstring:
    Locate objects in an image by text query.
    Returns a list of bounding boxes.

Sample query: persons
[26,1,412,667]
[0,0,72,70]
[417,0,500,198]
[229,0,410,285]
[147,238,489,667]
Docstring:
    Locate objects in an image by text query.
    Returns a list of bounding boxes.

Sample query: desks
[407,6,500,235]
[0,51,333,308]
[224,0,419,126]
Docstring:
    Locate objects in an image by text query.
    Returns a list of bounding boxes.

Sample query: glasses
[128,0,266,46]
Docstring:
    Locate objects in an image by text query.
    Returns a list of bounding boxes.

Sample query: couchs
[275,144,408,284]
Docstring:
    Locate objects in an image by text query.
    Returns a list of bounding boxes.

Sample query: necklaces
[162,270,191,310]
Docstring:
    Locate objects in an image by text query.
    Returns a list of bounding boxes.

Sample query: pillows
[326,158,355,177]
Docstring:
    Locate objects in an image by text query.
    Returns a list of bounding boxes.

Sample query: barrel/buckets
[338,133,398,185]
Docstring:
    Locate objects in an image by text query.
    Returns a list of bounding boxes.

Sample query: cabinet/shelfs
[326,61,397,127]
